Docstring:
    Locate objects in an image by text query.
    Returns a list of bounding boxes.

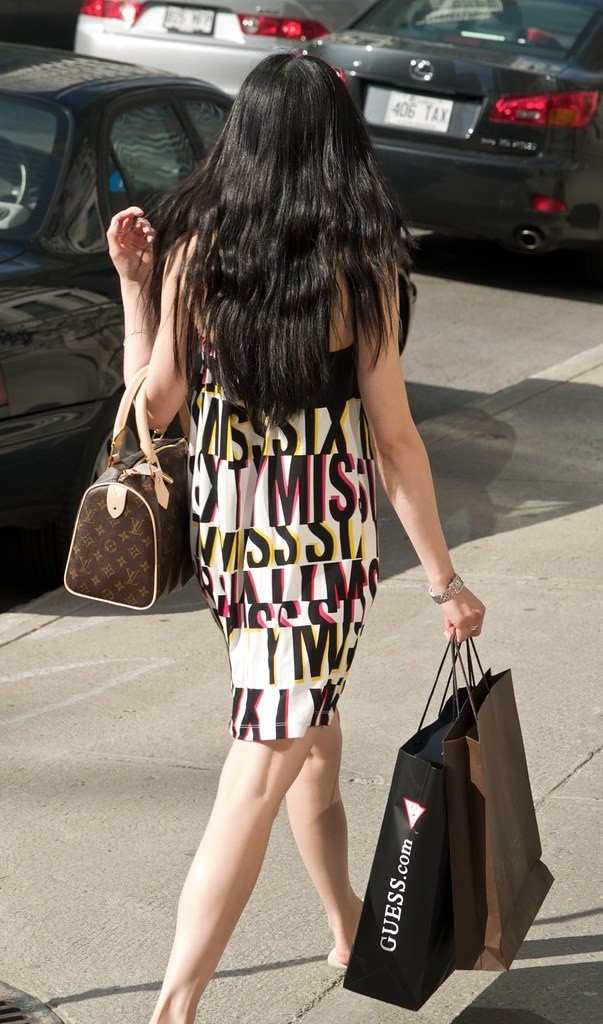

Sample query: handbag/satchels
[343,634,455,1012]
[443,630,554,971]
[63,363,195,610]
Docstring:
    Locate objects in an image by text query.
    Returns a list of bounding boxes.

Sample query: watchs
[429,574,464,604]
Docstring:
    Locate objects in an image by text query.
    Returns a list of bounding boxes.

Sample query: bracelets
[123,331,157,346]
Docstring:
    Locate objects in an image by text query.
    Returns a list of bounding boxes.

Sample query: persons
[106,54,485,1024]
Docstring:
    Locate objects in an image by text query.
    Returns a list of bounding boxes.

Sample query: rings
[471,625,479,631]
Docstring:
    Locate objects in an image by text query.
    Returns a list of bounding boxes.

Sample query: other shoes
[327,947,348,970]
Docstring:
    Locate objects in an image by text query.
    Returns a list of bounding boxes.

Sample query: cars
[73,1,377,100]
[0,39,412,600]
[295,0,602,291]
